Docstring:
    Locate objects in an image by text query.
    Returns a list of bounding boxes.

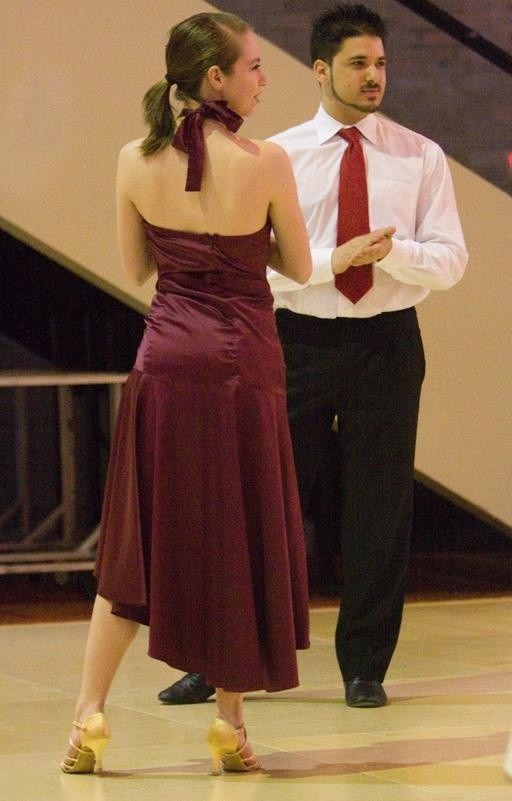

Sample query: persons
[157,2,471,708]
[60,9,315,774]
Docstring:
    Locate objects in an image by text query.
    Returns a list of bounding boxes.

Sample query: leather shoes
[158,671,216,704]
[345,677,387,708]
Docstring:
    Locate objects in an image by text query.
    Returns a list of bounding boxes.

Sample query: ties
[334,127,373,304]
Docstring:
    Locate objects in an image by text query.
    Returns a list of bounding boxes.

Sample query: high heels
[60,713,111,775]
[205,716,266,776]
